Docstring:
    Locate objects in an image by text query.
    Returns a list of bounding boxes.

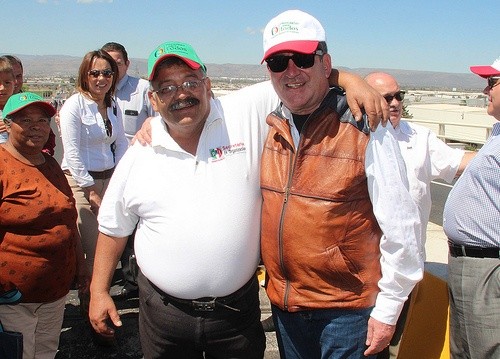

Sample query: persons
[365,71,478,347]
[441,57,500,359]
[1,55,24,93]
[89,41,391,359]
[0,91,89,359]
[132,9,425,359]
[59,49,131,321]
[103,42,159,294]
[0,57,56,156]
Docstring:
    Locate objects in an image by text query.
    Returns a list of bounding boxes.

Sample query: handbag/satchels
[0,289,22,359]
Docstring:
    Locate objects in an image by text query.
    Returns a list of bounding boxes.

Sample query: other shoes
[261,315,275,331]
[80,294,91,316]
[256,268,266,287]
[115,289,136,300]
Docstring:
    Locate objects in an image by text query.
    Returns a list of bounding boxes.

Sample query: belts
[448,239,500,258]
[151,281,256,312]
[64,165,116,180]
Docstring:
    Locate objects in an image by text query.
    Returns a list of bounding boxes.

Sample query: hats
[148,41,208,82]
[2,92,58,124]
[470,59,500,78]
[260,10,327,64]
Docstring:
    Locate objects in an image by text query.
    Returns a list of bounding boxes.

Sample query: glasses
[488,76,500,88]
[88,69,114,78]
[153,76,206,98]
[267,50,324,73]
[385,90,406,102]
